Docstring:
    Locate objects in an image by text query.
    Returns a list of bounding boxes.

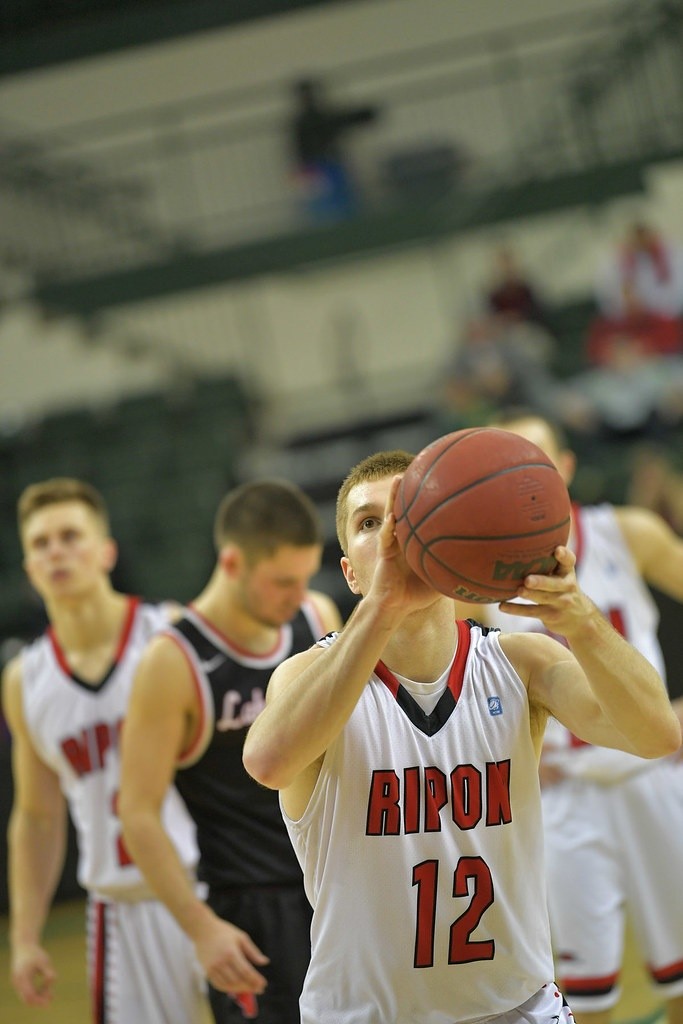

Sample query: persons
[243,450,683,1024]
[0,77,683,1024]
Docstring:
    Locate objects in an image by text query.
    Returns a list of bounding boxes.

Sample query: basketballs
[392,427,573,606]
